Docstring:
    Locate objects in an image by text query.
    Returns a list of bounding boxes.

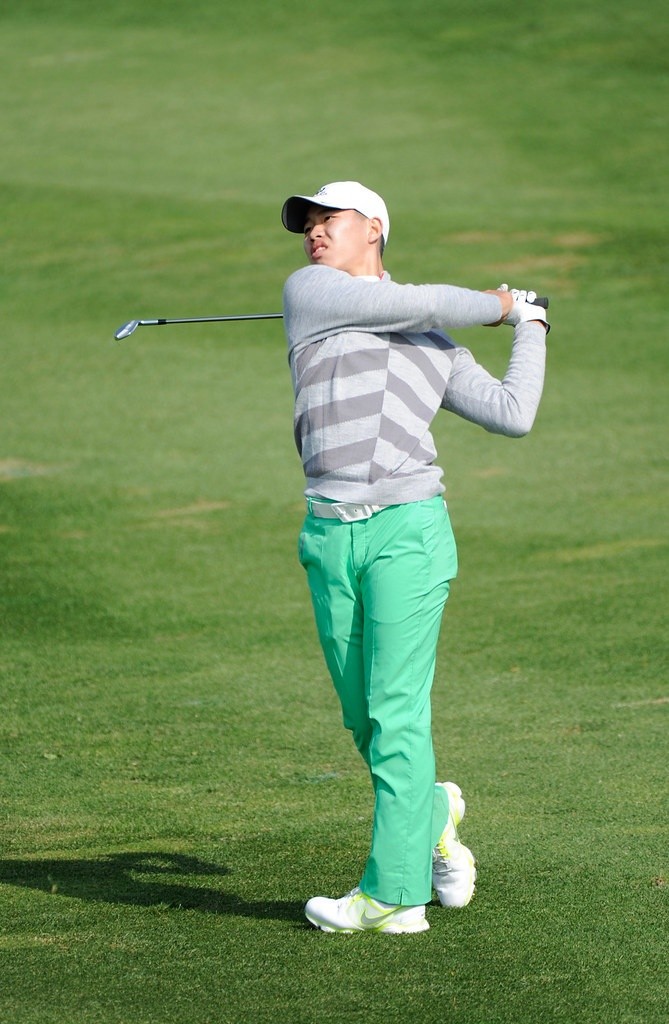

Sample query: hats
[280,181,390,245]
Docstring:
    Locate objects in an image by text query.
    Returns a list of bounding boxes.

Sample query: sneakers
[431,782,477,909]
[303,886,430,934]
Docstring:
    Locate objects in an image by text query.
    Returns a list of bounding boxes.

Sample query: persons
[281,181,548,935]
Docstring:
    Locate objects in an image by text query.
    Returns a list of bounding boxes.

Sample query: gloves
[496,283,551,335]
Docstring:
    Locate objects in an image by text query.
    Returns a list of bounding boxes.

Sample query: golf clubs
[112,296,552,343]
